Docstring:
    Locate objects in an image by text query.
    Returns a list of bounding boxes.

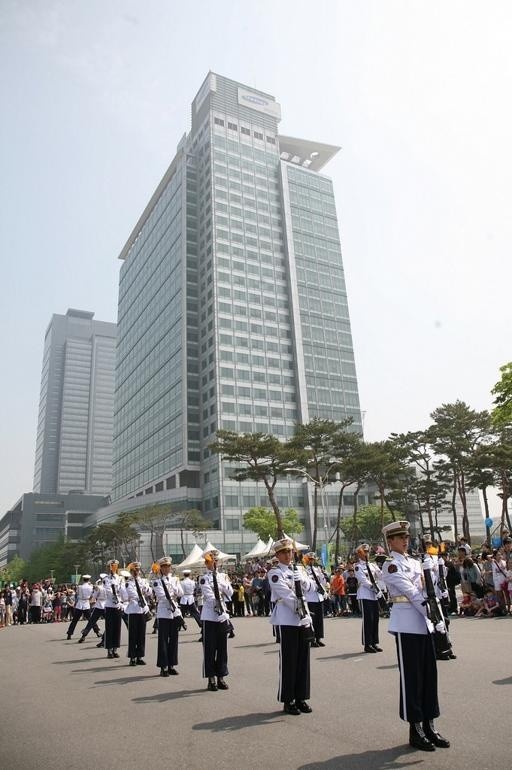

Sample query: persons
[0,521,512,751]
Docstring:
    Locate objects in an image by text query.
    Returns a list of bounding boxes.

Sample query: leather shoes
[217,677,228,690]
[284,702,301,715]
[208,677,218,691]
[311,638,319,647]
[228,631,235,638]
[409,722,435,751]
[295,699,312,713]
[108,649,113,658]
[183,625,187,631]
[152,628,156,634]
[113,648,120,658]
[316,638,325,646]
[449,653,456,658]
[95,640,104,647]
[199,635,203,641]
[160,667,169,677]
[373,644,383,653]
[79,636,85,643]
[96,632,102,637]
[422,720,451,748]
[365,645,377,653]
[130,658,137,666]
[67,635,71,640]
[168,666,178,675]
[437,656,449,660]
[136,657,146,665]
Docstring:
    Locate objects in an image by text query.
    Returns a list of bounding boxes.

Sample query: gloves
[375,590,383,598]
[293,570,305,584]
[300,615,312,628]
[173,608,181,617]
[143,605,149,614]
[420,558,432,570]
[438,557,445,565]
[217,612,229,623]
[116,602,122,609]
[436,620,446,635]
[136,576,142,583]
[438,589,449,599]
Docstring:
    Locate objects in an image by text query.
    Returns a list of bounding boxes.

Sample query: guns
[75,586,78,605]
[111,584,128,627]
[213,562,234,638]
[293,563,314,641]
[161,579,187,631]
[309,562,325,595]
[366,556,389,611]
[417,534,452,656]
[438,545,449,606]
[134,577,153,621]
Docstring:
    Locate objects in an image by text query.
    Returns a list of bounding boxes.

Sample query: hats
[107,559,119,565]
[355,544,370,554]
[304,551,315,559]
[82,574,91,579]
[100,573,108,578]
[128,561,141,568]
[158,556,172,566]
[182,570,191,575]
[201,549,219,561]
[381,520,410,537]
[120,571,132,577]
[272,538,293,553]
